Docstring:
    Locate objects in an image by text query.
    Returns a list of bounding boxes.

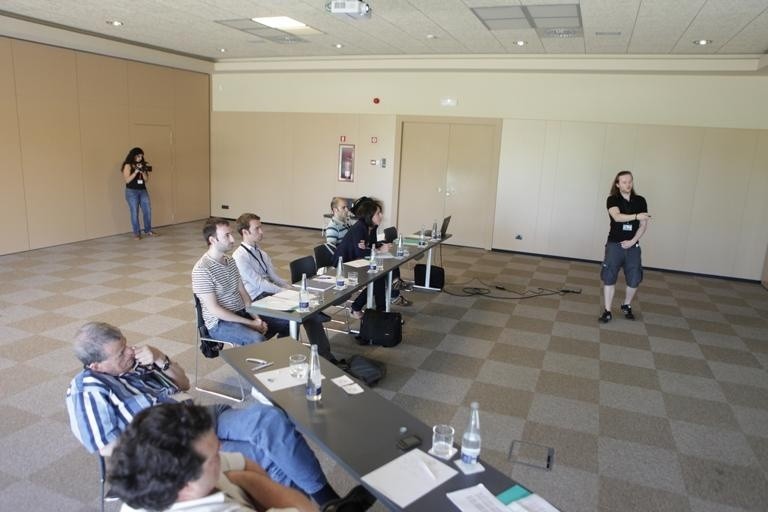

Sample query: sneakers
[322,485,375,512]
[599,310,613,321]
[350,310,364,319]
[622,305,634,319]
[392,280,412,291]
[392,295,411,306]
[145,231,159,236]
[134,234,141,240]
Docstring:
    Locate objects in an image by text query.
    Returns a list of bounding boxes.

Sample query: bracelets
[635,213,637,221]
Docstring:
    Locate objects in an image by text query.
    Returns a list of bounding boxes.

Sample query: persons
[233,212,351,370]
[598,172,650,322]
[123,148,156,240]
[192,219,268,344]
[330,197,388,321]
[66,322,342,504]
[108,405,377,511]
[321,197,414,306]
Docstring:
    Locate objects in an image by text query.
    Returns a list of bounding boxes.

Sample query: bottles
[305,345,323,402]
[460,402,482,471]
[298,274,310,313]
[396,233,404,257]
[335,257,345,291]
[368,244,378,273]
[430,223,437,240]
[417,225,426,247]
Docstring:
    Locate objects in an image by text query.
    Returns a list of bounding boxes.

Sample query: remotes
[647,216,650,217]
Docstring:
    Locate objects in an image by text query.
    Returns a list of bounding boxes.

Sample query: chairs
[95,452,108,512]
[314,242,356,323]
[289,256,351,338]
[384,226,398,243]
[322,198,355,236]
[194,294,279,402]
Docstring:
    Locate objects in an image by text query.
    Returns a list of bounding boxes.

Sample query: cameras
[136,163,152,173]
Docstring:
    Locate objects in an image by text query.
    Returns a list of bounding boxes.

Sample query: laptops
[413,217,451,240]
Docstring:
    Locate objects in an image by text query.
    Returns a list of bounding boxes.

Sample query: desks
[246,227,453,343]
[217,336,563,512]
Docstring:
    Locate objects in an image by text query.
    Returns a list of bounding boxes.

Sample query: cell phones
[395,435,422,453]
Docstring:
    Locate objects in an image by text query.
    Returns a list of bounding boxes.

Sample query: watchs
[159,354,171,372]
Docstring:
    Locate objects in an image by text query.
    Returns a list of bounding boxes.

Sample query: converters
[496,286,505,290]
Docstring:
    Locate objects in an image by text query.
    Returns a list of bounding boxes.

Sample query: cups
[424,239,429,247]
[432,424,454,457]
[347,272,358,285]
[288,354,306,379]
[374,256,382,271]
[402,248,409,257]
[436,232,441,241]
[308,291,319,308]
[419,225,425,237]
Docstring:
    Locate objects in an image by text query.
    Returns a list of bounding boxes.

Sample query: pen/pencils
[423,460,435,481]
[249,360,275,368]
[246,356,266,364]
[316,275,330,279]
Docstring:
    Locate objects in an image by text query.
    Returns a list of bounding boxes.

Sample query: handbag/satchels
[344,355,385,386]
[358,310,403,348]
